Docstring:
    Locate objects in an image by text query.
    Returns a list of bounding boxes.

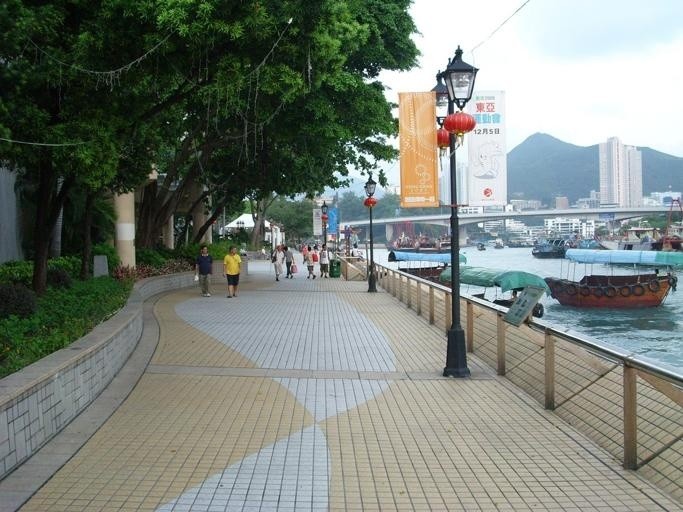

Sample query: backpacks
[312,252,319,261]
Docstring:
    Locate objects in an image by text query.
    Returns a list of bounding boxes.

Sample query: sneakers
[201,293,211,297]
[275,275,328,281]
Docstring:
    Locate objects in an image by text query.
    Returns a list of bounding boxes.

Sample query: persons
[271,243,332,281]
[222,246,242,298]
[352,243,364,261]
[194,245,213,297]
[508,290,518,305]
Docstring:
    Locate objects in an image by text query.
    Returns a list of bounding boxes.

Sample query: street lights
[364,175,377,292]
[322,201,328,245]
[430,45,479,378]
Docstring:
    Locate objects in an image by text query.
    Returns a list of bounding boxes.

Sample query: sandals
[227,294,237,298]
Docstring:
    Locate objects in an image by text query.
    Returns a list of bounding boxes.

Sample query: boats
[386,226,683,317]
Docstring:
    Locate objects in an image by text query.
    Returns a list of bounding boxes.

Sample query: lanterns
[363,197,377,209]
[437,111,477,157]
[321,214,328,221]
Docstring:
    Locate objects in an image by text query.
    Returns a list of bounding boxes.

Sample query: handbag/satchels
[291,265,298,273]
[272,256,277,263]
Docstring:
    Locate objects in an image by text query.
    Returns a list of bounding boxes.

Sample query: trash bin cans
[329,259,341,278]
